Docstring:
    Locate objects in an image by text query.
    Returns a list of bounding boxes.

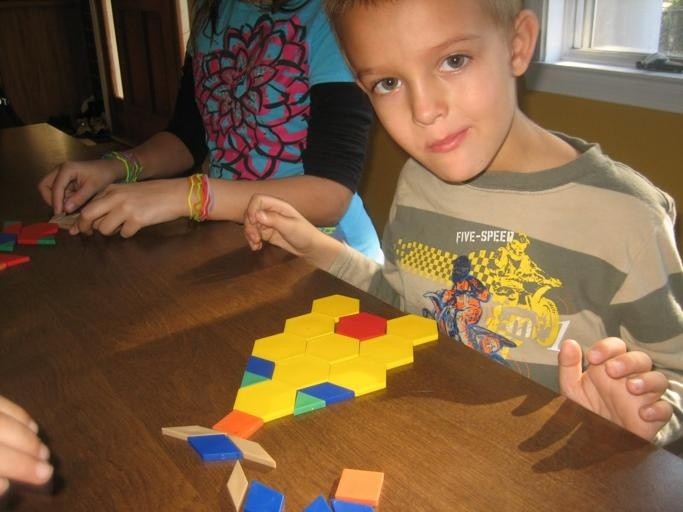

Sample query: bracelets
[100,148,144,184]
[188,171,214,222]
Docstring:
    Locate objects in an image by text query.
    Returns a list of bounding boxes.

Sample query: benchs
[357,90,683,250]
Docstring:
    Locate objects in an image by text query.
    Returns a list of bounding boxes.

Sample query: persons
[37,2,386,269]
[0,394,55,498]
[246,0,683,447]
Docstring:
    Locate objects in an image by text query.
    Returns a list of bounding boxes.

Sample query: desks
[1,122,683,512]
